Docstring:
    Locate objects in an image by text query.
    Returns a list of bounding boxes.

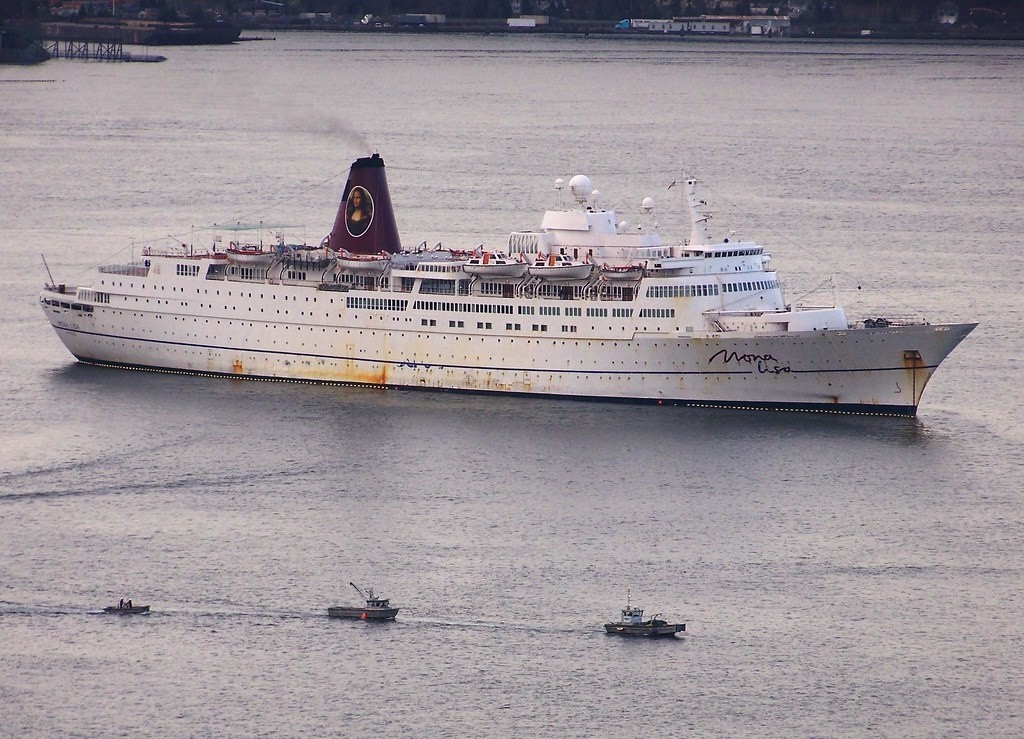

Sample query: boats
[328,582,400,620]
[463,251,528,279]
[39,150,980,419]
[334,247,388,271]
[528,251,592,280]
[102,605,151,615]
[601,262,643,281]
[224,239,275,264]
[604,587,686,636]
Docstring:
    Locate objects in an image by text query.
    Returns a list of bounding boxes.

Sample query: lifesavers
[268,277,274,285]
[145,250,148,255]
[245,243,251,248]
